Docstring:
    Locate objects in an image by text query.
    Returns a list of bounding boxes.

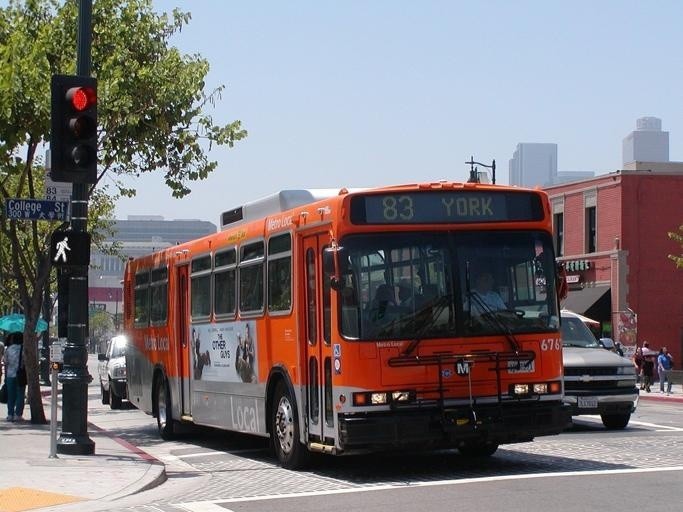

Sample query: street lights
[463,153,497,185]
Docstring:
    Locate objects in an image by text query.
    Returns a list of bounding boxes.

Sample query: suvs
[517,307,639,433]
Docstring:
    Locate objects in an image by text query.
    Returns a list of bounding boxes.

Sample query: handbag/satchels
[16,368,28,385]
[0,383,9,404]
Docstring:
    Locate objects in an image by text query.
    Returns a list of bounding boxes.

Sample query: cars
[96,334,128,411]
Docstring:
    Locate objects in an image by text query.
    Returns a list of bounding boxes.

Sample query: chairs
[419,284,444,300]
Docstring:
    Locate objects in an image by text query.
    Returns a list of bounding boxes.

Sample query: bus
[121,177,572,468]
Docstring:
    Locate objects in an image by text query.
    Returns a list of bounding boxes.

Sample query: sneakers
[5,414,23,421]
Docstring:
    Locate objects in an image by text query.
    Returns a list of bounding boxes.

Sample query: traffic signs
[6,197,68,224]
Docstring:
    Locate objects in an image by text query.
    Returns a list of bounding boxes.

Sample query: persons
[233,322,256,383]
[3,331,26,421]
[632,341,674,395]
[191,328,199,367]
[0,342,4,384]
[463,269,509,325]
[394,276,424,315]
[4,334,14,347]
[614,341,624,357]
[367,281,396,320]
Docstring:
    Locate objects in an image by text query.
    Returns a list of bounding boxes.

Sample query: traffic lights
[46,229,91,268]
[48,73,99,185]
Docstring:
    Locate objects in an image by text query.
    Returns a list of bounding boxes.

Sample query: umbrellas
[0,312,48,332]
[560,308,600,329]
